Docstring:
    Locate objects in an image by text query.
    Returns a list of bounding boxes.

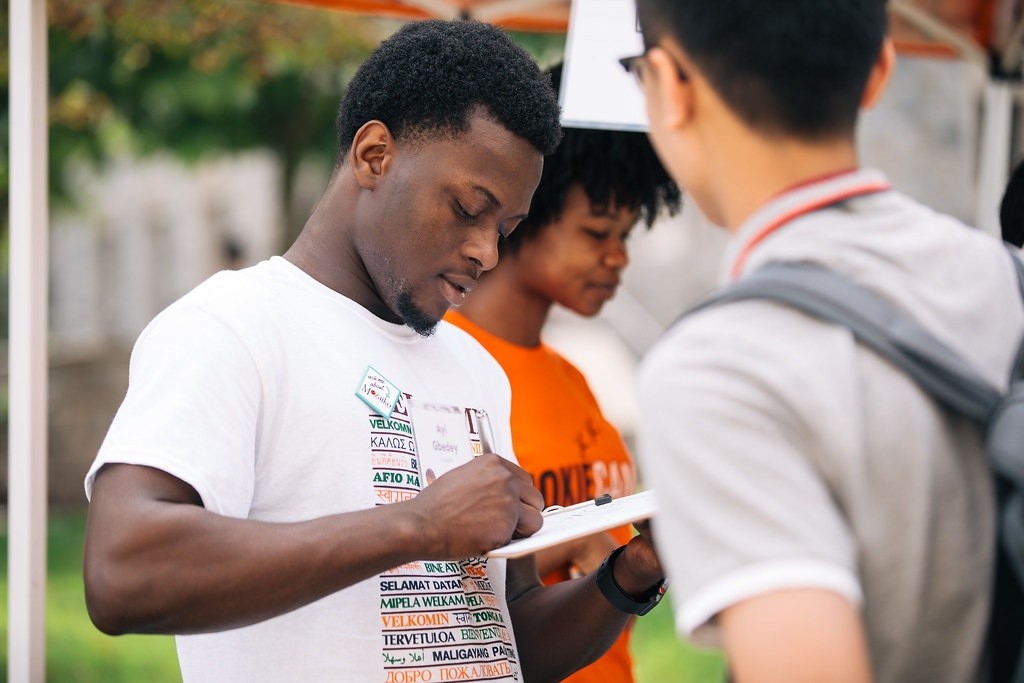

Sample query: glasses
[616,55,690,106]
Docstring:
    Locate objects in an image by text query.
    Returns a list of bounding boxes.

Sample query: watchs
[596,544,671,616]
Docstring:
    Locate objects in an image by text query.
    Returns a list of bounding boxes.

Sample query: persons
[448,62,682,683]
[82,13,666,683]
[624,0,1024,683]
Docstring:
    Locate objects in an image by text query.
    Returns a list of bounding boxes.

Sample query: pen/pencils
[476,407,496,454]
